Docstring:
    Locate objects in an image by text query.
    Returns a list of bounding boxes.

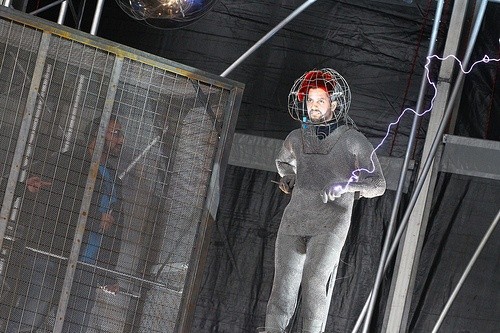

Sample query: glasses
[108,129,123,137]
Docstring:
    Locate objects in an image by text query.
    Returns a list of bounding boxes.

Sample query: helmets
[297,71,342,100]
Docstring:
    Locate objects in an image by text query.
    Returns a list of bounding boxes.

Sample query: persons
[0,177,54,213]
[5,115,124,333]
[264,68,386,332]
